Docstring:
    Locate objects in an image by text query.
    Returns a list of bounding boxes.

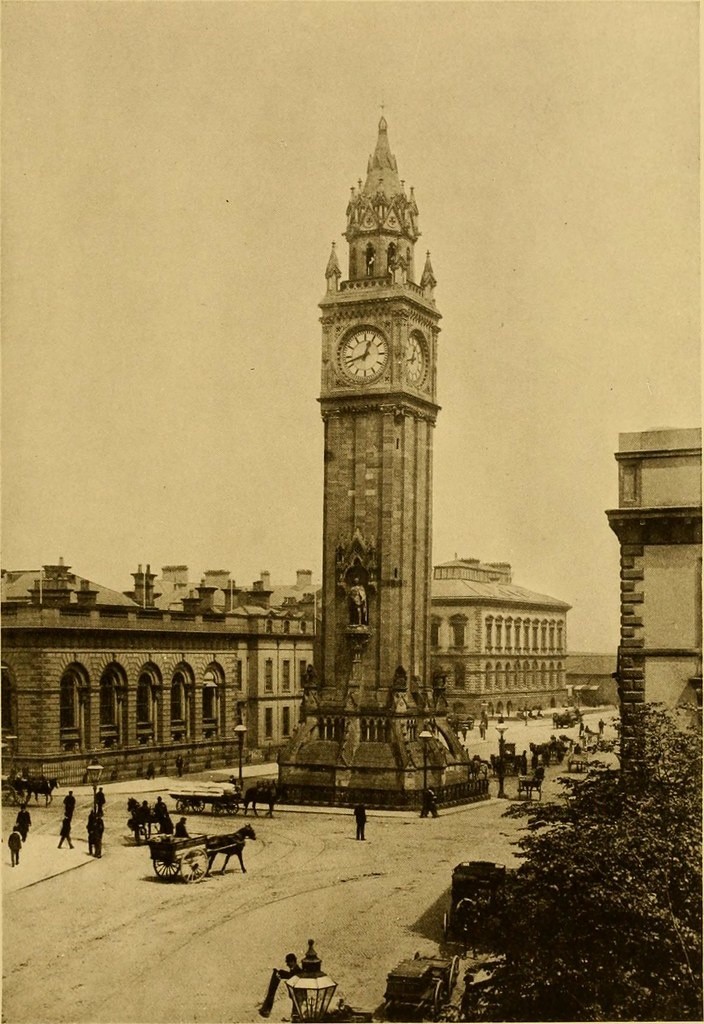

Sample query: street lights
[419,730,433,796]
[234,723,249,792]
[87,757,104,815]
[495,721,510,799]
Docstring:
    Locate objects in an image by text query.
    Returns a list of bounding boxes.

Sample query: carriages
[1,776,60,809]
[145,822,257,883]
[169,780,287,818]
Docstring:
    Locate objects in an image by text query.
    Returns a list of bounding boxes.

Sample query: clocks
[342,330,389,382]
[405,337,425,384]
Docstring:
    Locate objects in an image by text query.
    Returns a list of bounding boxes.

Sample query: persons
[85,811,105,857]
[521,749,528,775]
[350,574,369,625]
[599,718,606,734]
[16,804,32,842]
[229,775,236,785]
[304,664,317,682]
[273,954,306,1016]
[421,784,439,818]
[433,665,450,709]
[478,709,489,741]
[146,761,155,779]
[62,790,76,822]
[95,787,106,817]
[354,802,368,840]
[176,755,184,777]
[57,811,75,849]
[133,796,174,835]
[528,711,537,720]
[175,817,189,837]
[7,826,23,867]
[574,743,581,754]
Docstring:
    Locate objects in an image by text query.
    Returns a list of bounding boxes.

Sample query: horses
[205,824,255,876]
[25,778,60,806]
[243,784,281,817]
[127,797,144,835]
[529,742,551,759]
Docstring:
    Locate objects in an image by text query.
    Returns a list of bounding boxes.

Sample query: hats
[285,954,297,962]
